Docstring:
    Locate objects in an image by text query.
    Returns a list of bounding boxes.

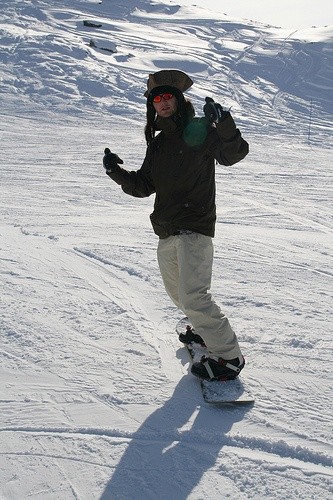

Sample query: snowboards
[175,316,255,406]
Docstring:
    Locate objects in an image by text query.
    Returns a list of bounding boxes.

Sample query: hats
[144,70,194,98]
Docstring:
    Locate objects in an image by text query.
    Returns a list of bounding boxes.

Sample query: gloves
[203,97,223,123]
[103,148,123,172]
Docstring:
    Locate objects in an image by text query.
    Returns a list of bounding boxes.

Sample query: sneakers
[191,355,245,379]
[179,330,207,347]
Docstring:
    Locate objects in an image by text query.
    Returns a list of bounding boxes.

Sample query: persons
[103,70,249,381]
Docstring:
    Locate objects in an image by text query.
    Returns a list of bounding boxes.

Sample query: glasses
[153,93,173,103]
